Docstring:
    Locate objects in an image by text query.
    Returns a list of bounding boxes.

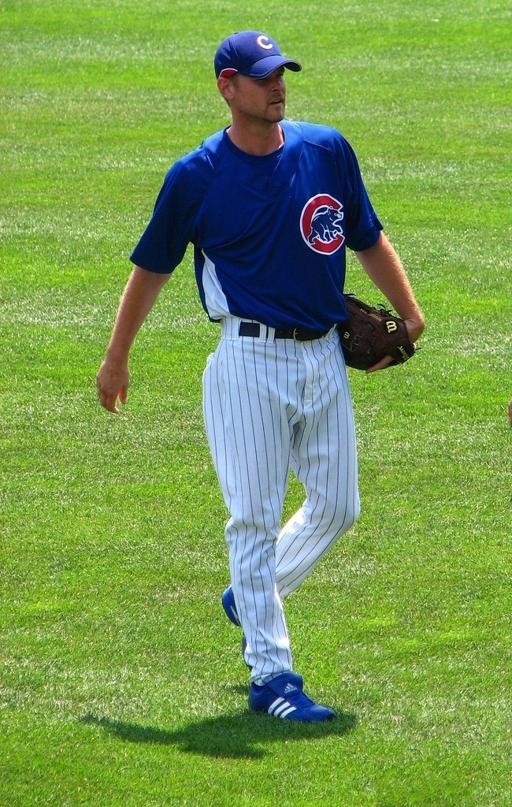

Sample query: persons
[87,28,427,726]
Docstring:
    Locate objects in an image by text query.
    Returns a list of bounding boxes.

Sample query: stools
[239,317,340,342]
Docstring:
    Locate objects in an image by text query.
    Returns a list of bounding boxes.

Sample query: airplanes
[338,294,416,370]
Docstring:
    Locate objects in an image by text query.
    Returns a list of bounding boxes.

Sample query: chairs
[248,671,338,722]
[221,581,260,671]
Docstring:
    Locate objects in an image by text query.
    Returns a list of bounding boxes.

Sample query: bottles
[214,30,302,79]
